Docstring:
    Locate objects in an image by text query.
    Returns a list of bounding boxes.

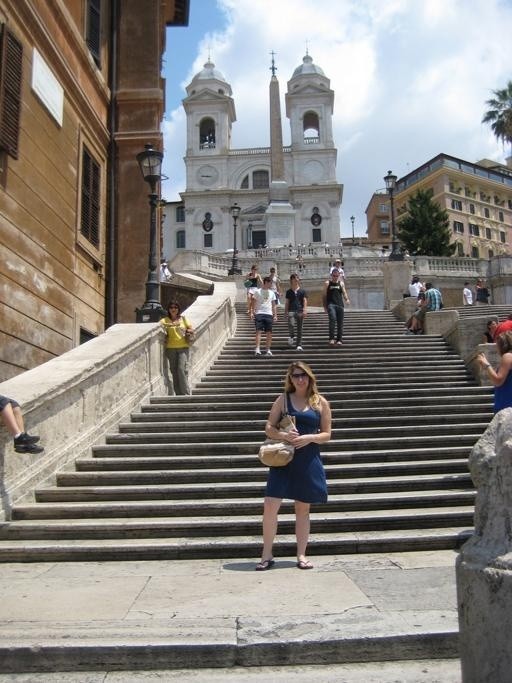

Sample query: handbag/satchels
[258,392,298,467]
[244,280,253,288]
[186,328,197,346]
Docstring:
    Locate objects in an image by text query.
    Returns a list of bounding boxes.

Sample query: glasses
[292,373,309,380]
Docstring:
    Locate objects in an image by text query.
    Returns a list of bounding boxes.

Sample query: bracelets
[482,362,491,369]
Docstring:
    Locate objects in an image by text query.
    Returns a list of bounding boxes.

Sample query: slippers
[255,557,275,571]
[296,557,314,570]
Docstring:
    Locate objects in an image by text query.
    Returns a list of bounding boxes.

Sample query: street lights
[227,201,243,276]
[136,139,169,322]
[349,215,356,244]
[382,170,404,260]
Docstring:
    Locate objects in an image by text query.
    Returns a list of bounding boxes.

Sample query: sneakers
[329,339,342,346]
[255,350,261,357]
[15,444,44,454]
[266,348,273,356]
[14,432,41,448]
[403,328,424,335]
[288,336,294,345]
[297,346,303,351]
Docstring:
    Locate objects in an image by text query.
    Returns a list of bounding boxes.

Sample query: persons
[329,258,348,290]
[155,261,174,283]
[244,237,350,257]
[403,247,410,260]
[403,274,491,334]
[240,258,310,356]
[474,319,512,415]
[483,319,497,344]
[255,358,333,569]
[159,298,197,398]
[505,313,512,321]
[322,268,351,345]
[379,246,388,258]
[0,390,44,453]
